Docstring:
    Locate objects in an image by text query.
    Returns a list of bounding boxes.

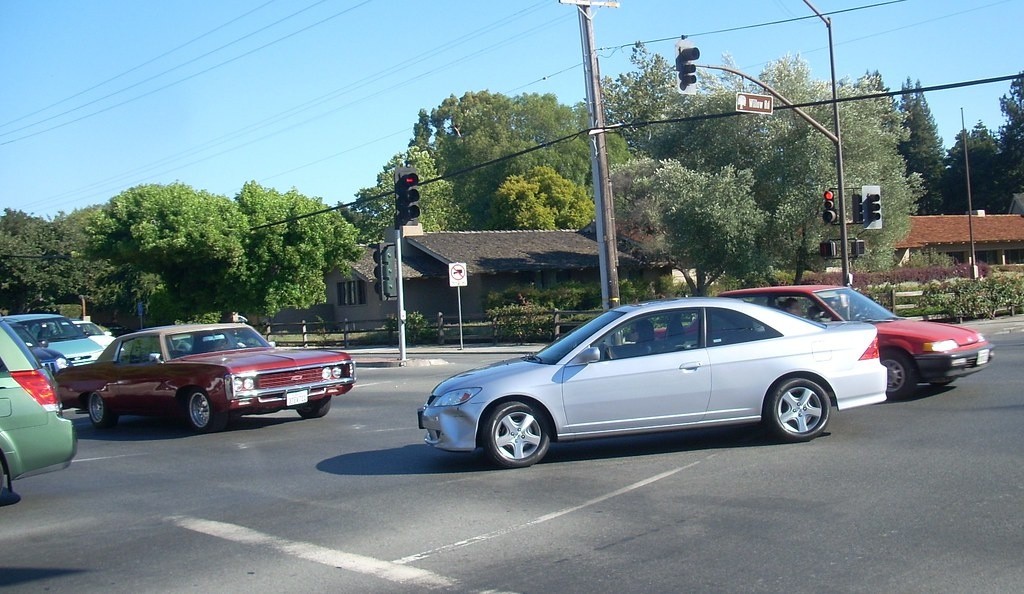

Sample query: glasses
[629,329,637,333]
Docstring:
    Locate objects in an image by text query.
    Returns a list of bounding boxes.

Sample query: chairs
[665,315,685,347]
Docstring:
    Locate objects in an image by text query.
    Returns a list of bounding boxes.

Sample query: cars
[417,297,888,470]
[53,325,358,433]
[0,315,118,495]
[653,286,998,402]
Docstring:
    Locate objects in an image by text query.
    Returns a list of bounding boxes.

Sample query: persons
[782,296,848,322]
[606,320,655,360]
[517,293,529,307]
[41,325,53,337]
[218,330,247,351]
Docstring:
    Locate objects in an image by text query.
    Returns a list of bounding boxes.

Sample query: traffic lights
[819,240,834,258]
[822,190,837,224]
[399,171,420,221]
[867,194,881,221]
[681,46,700,83]
